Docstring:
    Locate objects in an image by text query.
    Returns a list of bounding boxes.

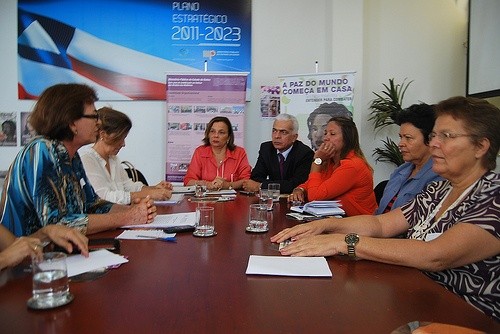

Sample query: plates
[193,231,217,238]
[24,292,75,310]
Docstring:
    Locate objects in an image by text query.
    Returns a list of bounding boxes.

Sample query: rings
[33,245,39,251]
[290,193,294,196]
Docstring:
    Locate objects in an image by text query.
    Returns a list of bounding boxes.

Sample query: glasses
[79,113,99,121]
[427,132,478,141]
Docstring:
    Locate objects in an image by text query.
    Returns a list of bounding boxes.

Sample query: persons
[241,114,316,197]
[305,101,354,152]
[286,116,378,218]
[0,82,158,252]
[269,94,500,324]
[78,106,173,206]
[182,116,251,191]
[369,101,448,220]
[21,113,39,143]
[0,120,17,144]
[0,223,90,275]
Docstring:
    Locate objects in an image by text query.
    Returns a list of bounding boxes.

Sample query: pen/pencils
[137,235,176,241]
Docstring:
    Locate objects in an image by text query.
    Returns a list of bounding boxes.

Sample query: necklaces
[212,151,227,166]
[413,187,462,240]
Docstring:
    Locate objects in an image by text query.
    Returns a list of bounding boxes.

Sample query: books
[289,199,347,219]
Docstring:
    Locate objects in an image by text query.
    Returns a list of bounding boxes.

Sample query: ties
[278,153,285,181]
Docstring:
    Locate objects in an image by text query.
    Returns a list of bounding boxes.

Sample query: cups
[259,189,273,209]
[268,183,280,200]
[245,227,269,232]
[249,204,268,230]
[30,252,71,304]
[195,206,214,234]
[195,179,206,196]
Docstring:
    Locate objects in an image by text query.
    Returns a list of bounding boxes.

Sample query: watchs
[344,233,360,258]
[228,182,232,190]
[310,158,324,166]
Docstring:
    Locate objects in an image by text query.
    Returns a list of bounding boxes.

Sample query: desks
[0,190,500,334]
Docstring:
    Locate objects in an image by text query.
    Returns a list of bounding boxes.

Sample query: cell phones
[163,225,195,233]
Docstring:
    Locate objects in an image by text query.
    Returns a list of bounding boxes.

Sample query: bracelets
[292,187,304,192]
